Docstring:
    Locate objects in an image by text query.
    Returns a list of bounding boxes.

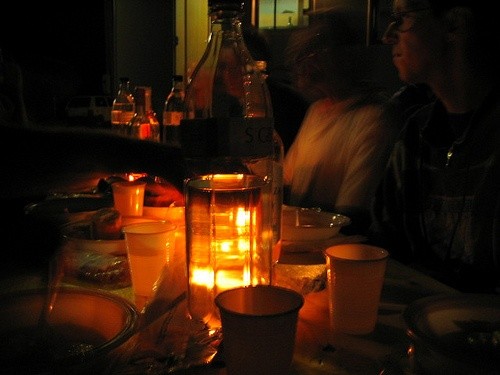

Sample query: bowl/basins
[60,219,127,281]
[0,287,138,375]
[405,293,500,375]
[283,208,349,238]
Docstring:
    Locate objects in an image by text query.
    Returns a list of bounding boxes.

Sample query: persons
[167,1,500,303]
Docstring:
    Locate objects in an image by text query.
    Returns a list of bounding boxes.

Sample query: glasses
[382,6,432,32]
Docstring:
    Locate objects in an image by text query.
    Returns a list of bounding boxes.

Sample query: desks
[38,203,461,375]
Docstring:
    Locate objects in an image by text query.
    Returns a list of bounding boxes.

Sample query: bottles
[110,79,135,132]
[183,0,271,333]
[164,79,195,147]
[243,60,284,265]
[128,86,160,140]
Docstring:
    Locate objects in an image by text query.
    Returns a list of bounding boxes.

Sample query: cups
[122,221,177,295]
[216,286,303,375]
[111,182,146,217]
[324,243,389,334]
